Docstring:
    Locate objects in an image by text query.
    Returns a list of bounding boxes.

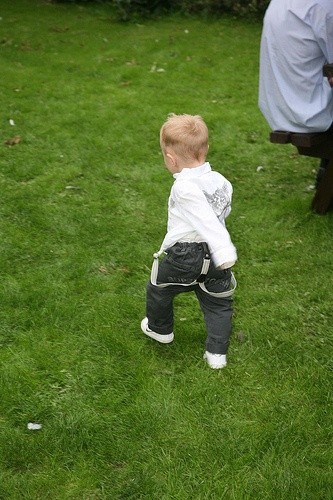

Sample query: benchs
[270,131,333,216]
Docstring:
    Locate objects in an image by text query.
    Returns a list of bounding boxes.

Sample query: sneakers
[203,351,226,369]
[141,317,174,343]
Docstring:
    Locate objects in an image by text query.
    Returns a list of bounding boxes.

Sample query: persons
[258,0,333,215]
[141,112,238,369]
